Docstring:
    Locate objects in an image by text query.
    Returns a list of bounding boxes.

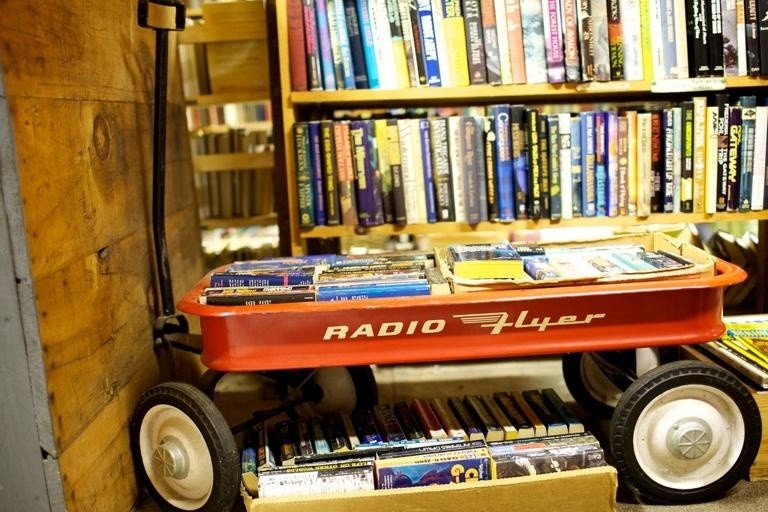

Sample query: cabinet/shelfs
[269,0,767,319]
[176,1,293,273]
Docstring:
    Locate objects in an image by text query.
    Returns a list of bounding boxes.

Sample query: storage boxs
[663,315,767,483]
[241,387,620,511]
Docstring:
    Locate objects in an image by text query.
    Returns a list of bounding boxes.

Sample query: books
[240,386,606,498]
[287,2,768,91]
[201,227,280,277]
[179,16,213,98]
[200,230,760,308]
[191,122,276,219]
[688,313,768,390]
[291,95,768,227]
